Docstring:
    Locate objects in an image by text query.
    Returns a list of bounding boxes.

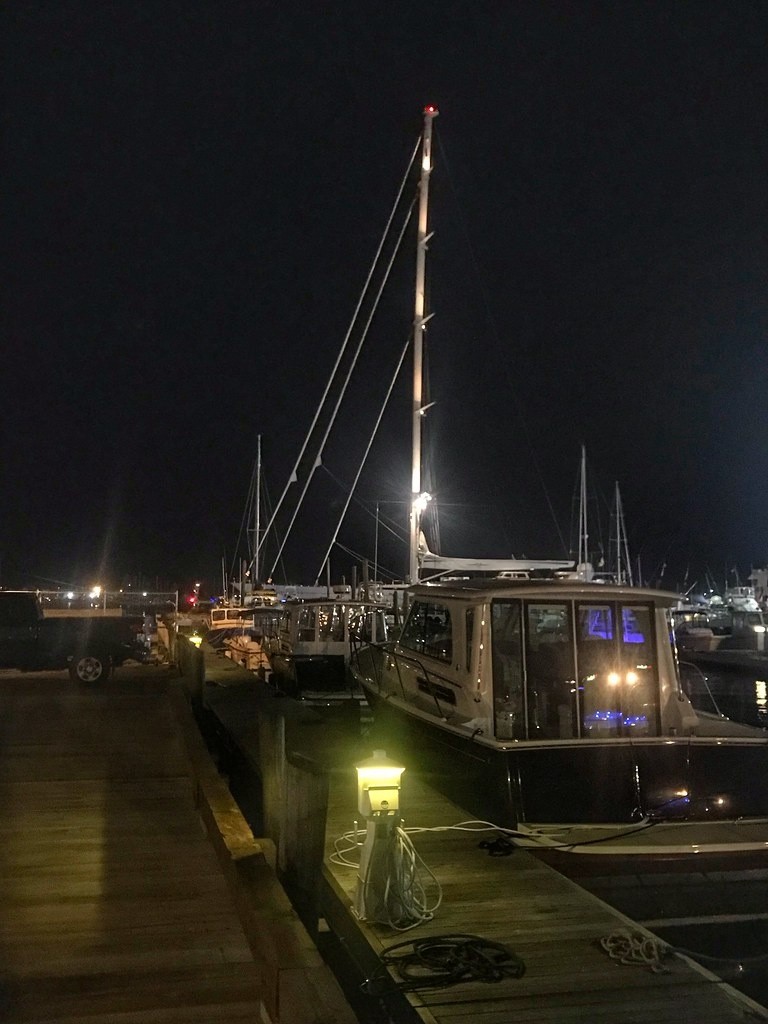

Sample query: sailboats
[203,103,768,728]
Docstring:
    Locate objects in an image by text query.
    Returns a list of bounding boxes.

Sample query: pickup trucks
[0,589,150,689]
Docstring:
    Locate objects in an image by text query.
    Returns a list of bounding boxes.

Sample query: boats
[350,438,768,823]
